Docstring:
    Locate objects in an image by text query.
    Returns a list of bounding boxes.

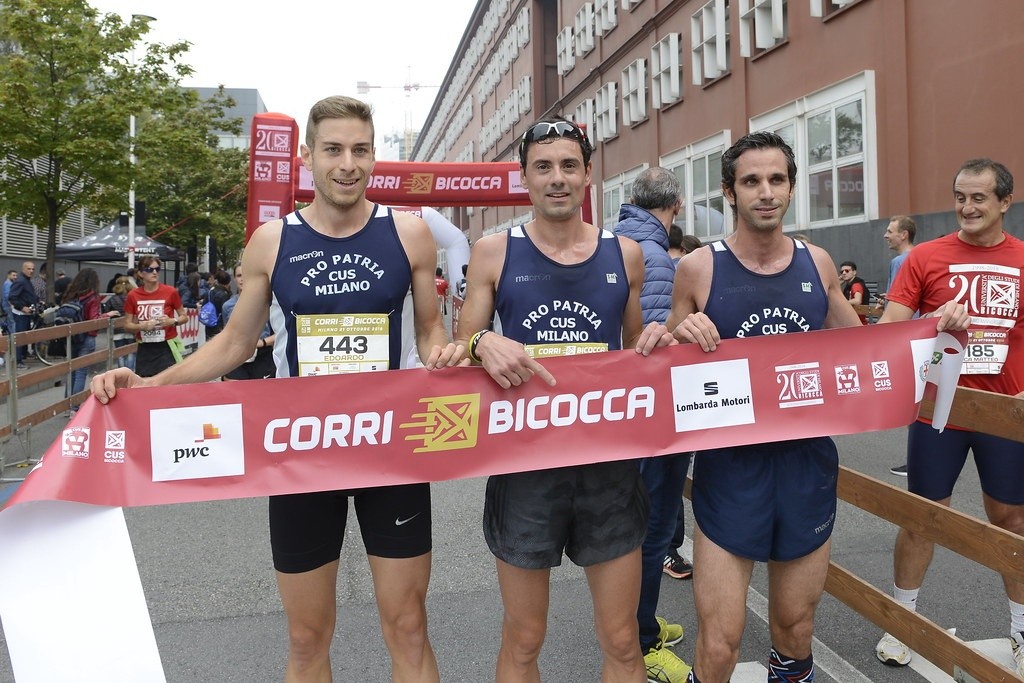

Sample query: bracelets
[924,312,930,319]
[257,337,266,349]
[469,330,492,363]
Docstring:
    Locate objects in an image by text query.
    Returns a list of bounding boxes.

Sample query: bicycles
[0,300,72,366]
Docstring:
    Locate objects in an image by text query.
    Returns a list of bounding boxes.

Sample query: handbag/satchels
[198,290,224,326]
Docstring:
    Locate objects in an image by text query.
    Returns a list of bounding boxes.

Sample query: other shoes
[63,409,77,419]
[16,362,30,371]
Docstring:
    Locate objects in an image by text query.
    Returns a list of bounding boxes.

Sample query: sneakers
[876,630,911,664]
[643,630,692,683]
[662,552,693,578]
[652,616,684,650]
[1011,630,1024,676]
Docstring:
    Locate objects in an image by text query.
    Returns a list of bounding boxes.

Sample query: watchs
[173,318,178,327]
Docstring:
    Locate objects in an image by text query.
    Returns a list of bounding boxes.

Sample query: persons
[456,264,468,296]
[840,261,870,325]
[178,265,277,382]
[877,160,1024,680]
[89,97,470,682]
[664,132,971,683]
[454,118,679,682]
[874,215,918,475]
[124,257,188,378]
[435,268,448,302]
[0,261,144,419]
[610,166,699,683]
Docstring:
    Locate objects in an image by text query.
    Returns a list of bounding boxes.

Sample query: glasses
[841,268,852,274]
[521,120,587,162]
[140,266,160,272]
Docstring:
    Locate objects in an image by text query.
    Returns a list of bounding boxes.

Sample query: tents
[55,220,187,275]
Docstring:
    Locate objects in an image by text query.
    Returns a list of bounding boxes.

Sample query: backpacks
[55,293,94,344]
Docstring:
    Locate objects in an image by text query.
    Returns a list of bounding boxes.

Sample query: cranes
[356,65,443,162]
